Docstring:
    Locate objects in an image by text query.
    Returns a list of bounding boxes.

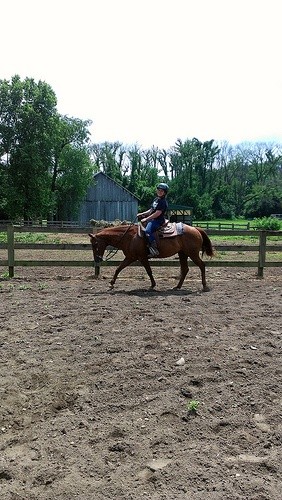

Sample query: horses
[88,222,213,291]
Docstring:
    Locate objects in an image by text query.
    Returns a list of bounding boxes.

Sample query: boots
[148,239,159,257]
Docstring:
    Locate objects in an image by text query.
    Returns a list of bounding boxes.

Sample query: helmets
[157,183,169,190]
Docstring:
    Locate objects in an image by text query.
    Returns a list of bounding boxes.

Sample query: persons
[137,183,169,257]
